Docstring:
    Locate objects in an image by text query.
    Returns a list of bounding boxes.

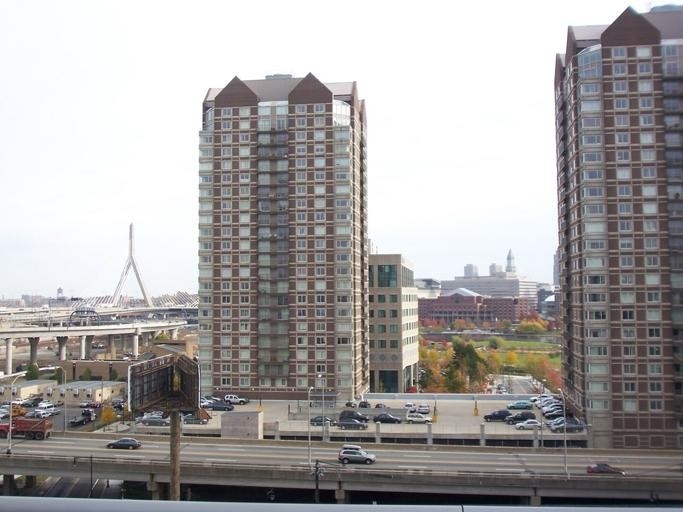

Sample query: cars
[587,463,625,476]
[342,445,360,450]
[79,400,103,408]
[311,415,337,426]
[346,402,385,408]
[0,397,60,418]
[508,401,532,410]
[200,393,246,411]
[338,410,368,429]
[405,402,430,413]
[140,411,207,425]
[106,438,141,449]
[112,398,127,410]
[530,394,583,432]
[484,409,512,421]
[374,413,401,424]
[516,420,543,430]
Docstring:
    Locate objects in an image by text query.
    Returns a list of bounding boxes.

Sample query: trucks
[0,416,51,440]
[70,409,96,425]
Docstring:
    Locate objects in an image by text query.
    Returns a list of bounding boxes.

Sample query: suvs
[339,449,376,464]
[505,411,536,424]
[407,413,432,424]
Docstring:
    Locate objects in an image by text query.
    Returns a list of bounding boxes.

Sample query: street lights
[539,378,547,446]
[557,387,567,472]
[305,385,314,464]
[318,374,324,426]
[6,372,26,449]
